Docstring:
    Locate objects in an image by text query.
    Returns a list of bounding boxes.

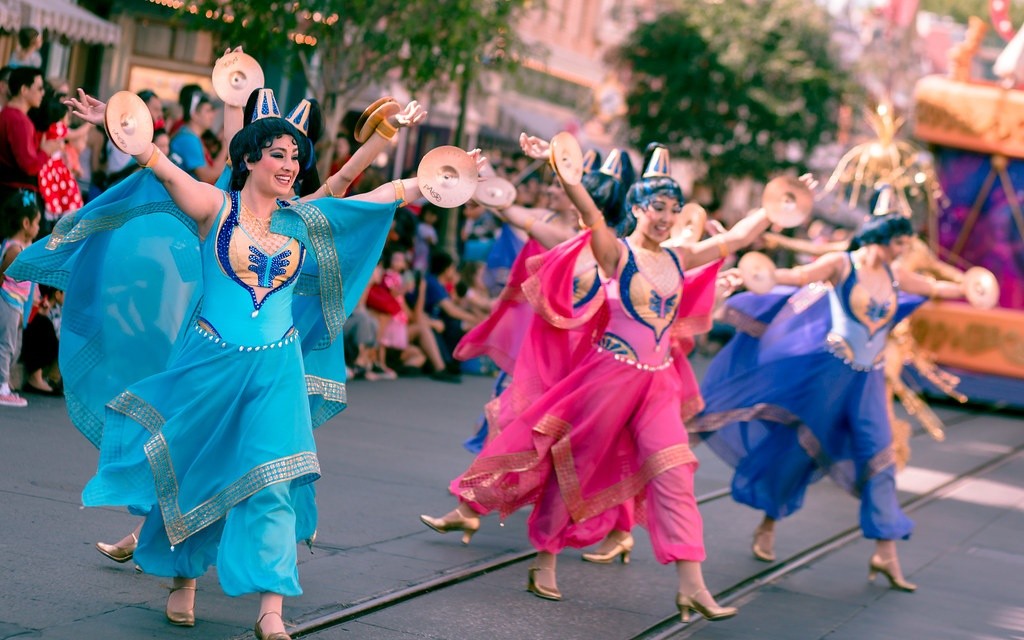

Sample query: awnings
[496,102,609,161]
[0,0,121,50]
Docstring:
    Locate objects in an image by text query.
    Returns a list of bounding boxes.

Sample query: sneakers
[0,394,28,406]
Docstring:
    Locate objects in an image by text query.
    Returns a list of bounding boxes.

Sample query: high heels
[419,508,480,544]
[753,525,776,561]
[676,588,738,623]
[95,532,145,572]
[255,611,292,640]
[527,567,561,601]
[868,554,917,591]
[161,584,199,627]
[582,533,634,564]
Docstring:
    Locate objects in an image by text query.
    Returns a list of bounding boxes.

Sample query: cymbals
[212,51,265,107]
[417,145,480,209]
[472,176,517,209]
[549,130,584,187]
[353,95,401,143]
[104,90,155,156]
[670,202,707,246]
[761,175,813,228]
[737,251,776,295]
[963,266,1000,309]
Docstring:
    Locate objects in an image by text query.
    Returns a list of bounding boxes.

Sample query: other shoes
[22,382,62,396]
[433,368,462,384]
[367,368,399,380]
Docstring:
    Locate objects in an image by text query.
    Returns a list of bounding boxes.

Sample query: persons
[97,95,429,574]
[4,85,489,640]
[0,64,553,407]
[419,132,818,623]
[210,44,319,198]
[751,176,967,593]
